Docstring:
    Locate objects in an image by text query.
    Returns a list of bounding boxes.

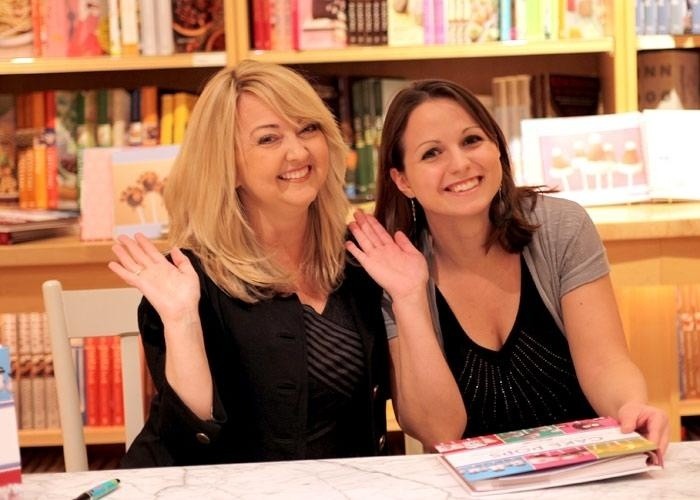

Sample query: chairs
[42,279,146,473]
[614,285,682,443]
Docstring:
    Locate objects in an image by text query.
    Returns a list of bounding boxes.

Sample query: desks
[0,442,700,499]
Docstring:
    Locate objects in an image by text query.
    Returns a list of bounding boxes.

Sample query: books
[390,1,613,47]
[1,0,224,59]
[434,414,664,496]
[68,336,122,426]
[480,72,601,145]
[248,1,389,48]
[0,90,106,245]
[109,86,201,147]
[635,1,698,34]
[348,80,420,201]
[642,110,699,203]
[1,311,60,429]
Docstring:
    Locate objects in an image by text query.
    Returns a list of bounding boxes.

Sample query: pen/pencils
[73,477,121,500]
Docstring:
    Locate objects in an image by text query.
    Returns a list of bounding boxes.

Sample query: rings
[134,267,146,275]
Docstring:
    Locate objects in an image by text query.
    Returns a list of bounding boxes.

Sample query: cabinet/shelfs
[0,0,700,449]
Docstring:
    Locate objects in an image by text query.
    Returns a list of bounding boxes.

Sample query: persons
[107,58,394,470]
[344,78,669,463]
